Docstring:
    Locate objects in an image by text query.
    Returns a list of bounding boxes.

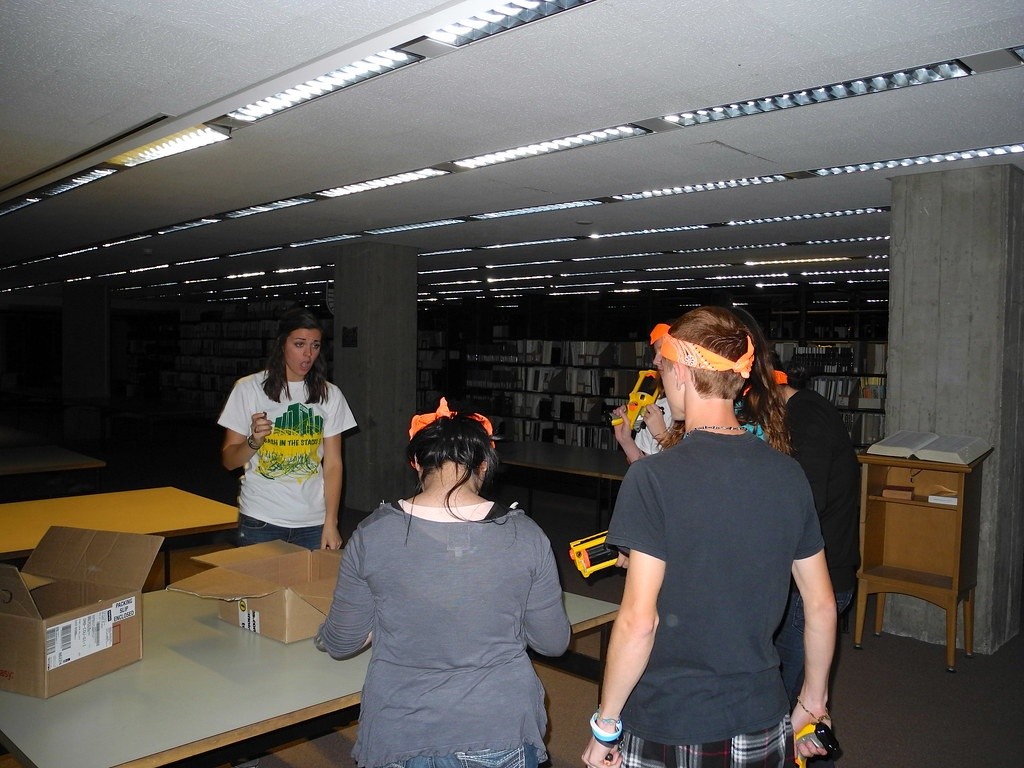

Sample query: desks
[0,588,622,768]
[0,487,240,589]
[0,444,106,475]
[496,441,631,533]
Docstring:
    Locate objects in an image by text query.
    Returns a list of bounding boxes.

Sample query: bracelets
[796,695,831,725]
[248,434,260,449]
[589,710,623,748]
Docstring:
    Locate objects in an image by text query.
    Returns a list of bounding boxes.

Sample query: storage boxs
[165,538,345,645]
[1,525,165,699]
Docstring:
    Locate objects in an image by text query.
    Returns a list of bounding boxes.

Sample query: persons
[313,409,571,768]
[216,309,357,550]
[579,305,859,768]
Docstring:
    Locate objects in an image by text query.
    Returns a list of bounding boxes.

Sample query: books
[407,325,885,476]
[864,428,992,466]
[117,296,334,426]
[881,485,915,501]
[927,490,958,505]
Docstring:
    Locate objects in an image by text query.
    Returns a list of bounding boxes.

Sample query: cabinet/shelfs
[125,301,886,499]
[853,446,995,673]
